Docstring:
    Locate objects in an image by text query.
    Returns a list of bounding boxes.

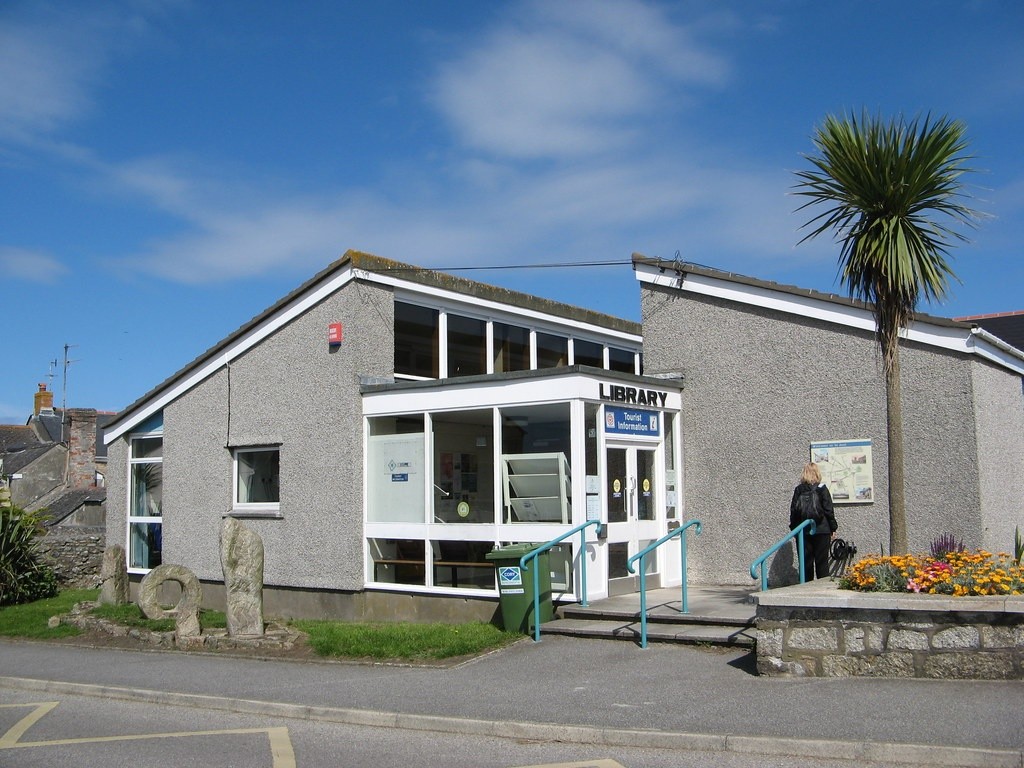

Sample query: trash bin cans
[486,542,555,634]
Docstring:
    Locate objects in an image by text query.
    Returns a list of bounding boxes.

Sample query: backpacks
[795,482,824,524]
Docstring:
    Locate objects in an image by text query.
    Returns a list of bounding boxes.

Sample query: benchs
[374,558,495,588]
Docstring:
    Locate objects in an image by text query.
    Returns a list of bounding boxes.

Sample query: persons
[787,462,838,582]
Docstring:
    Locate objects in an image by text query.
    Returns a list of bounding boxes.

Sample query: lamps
[506,416,528,427]
